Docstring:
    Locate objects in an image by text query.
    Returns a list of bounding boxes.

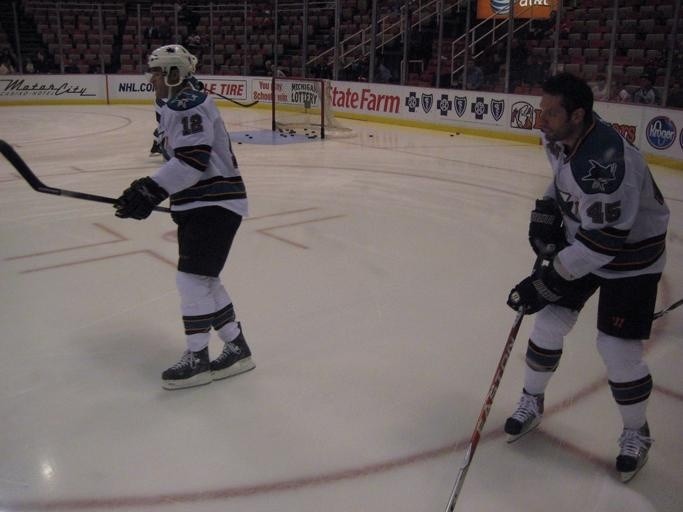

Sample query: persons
[503,71,669,472]
[1,2,682,109]
[111,44,255,381]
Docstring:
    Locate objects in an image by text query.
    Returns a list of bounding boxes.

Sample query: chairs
[0,2,683,113]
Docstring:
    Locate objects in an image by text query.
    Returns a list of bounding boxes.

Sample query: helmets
[148,45,197,88]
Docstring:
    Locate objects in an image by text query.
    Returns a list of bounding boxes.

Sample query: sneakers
[616,423,650,473]
[211,323,251,370]
[504,388,544,434]
[162,346,210,381]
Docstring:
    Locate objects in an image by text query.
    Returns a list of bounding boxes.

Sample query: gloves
[507,260,570,315]
[528,199,565,257]
[113,176,170,219]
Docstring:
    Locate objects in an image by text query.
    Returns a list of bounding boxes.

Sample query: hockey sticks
[0,139,170,213]
[203,87,258,107]
